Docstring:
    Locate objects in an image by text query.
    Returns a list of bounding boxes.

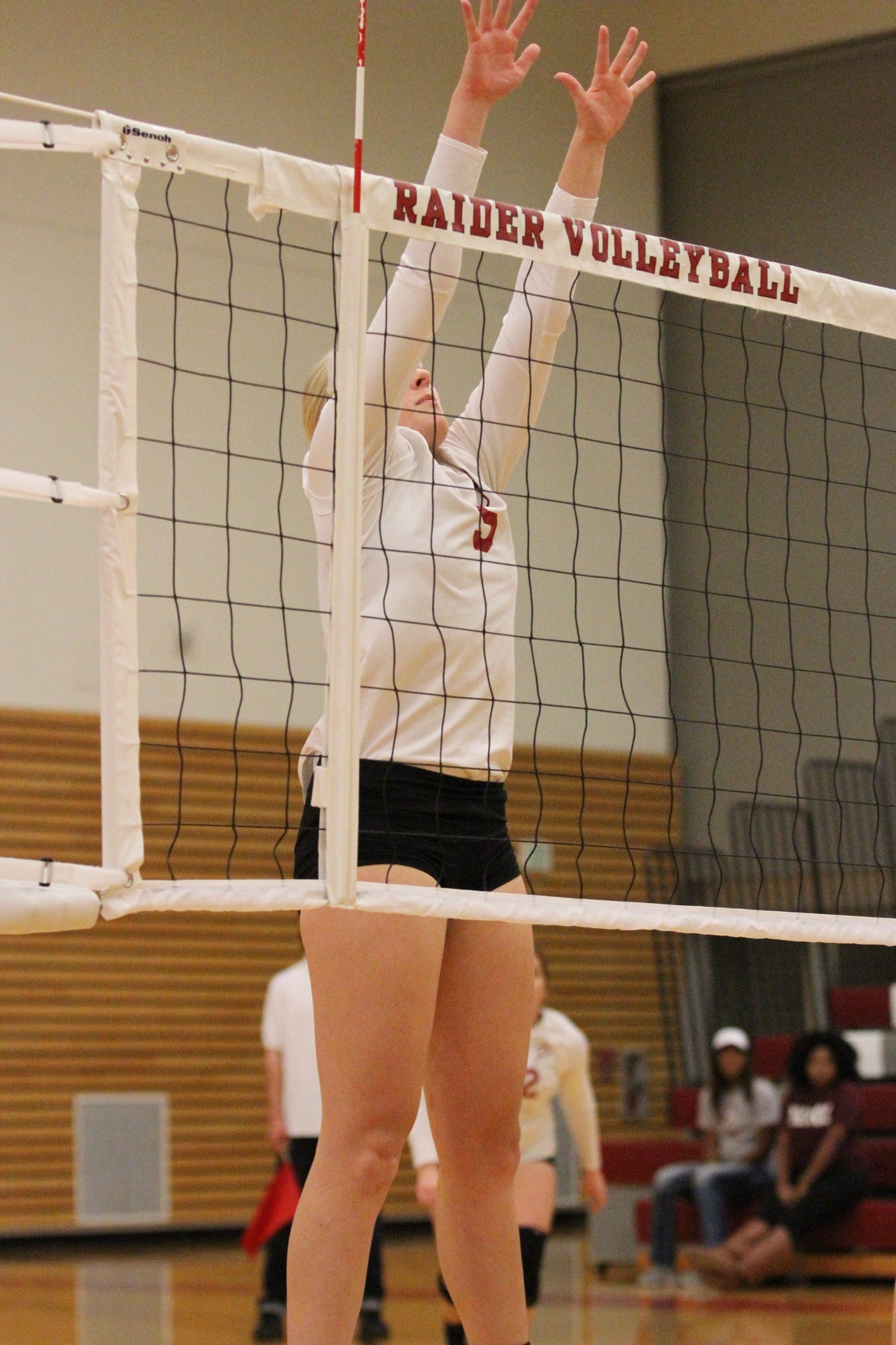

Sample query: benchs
[600,984,896,1280]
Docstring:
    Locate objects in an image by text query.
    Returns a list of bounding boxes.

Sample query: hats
[713,1026,751,1051]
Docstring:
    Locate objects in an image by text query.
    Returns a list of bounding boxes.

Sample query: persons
[632,1027,877,1298]
[289,0,657,1344]
[251,957,609,1345]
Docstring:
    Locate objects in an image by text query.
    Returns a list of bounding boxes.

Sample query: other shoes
[638,1266,674,1288]
[682,1246,743,1288]
[684,1271,708,1288]
[257,1288,282,1313]
[359,1281,386,1316]
